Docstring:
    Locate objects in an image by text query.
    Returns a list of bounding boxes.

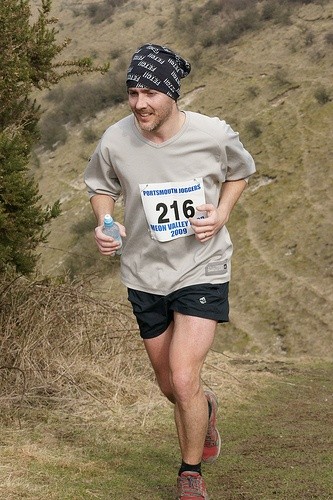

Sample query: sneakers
[201,390,221,465]
[177,471,208,500]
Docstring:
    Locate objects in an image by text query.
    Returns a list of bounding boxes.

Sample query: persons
[83,43,256,500]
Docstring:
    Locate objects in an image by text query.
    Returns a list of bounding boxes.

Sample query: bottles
[102,214,123,248]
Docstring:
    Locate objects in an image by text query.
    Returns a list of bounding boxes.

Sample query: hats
[126,43,191,101]
